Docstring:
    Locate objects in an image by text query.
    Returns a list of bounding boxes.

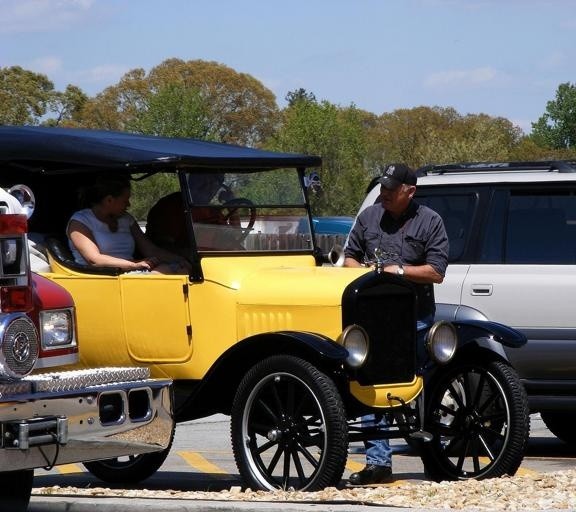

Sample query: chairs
[47,231,120,275]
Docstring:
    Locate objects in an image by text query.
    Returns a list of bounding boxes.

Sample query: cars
[0,194,174,507]
[3,128,541,493]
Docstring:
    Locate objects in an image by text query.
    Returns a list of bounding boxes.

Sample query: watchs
[398,265,405,280]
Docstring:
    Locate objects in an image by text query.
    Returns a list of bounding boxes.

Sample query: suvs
[297,211,353,236]
[337,162,576,448]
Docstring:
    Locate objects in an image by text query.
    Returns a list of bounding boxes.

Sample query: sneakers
[349,464,392,485]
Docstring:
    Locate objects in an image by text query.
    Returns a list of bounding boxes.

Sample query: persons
[341,162,450,482]
[64,174,194,276]
[143,163,248,277]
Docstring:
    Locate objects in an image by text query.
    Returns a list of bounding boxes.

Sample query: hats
[376,164,417,190]
